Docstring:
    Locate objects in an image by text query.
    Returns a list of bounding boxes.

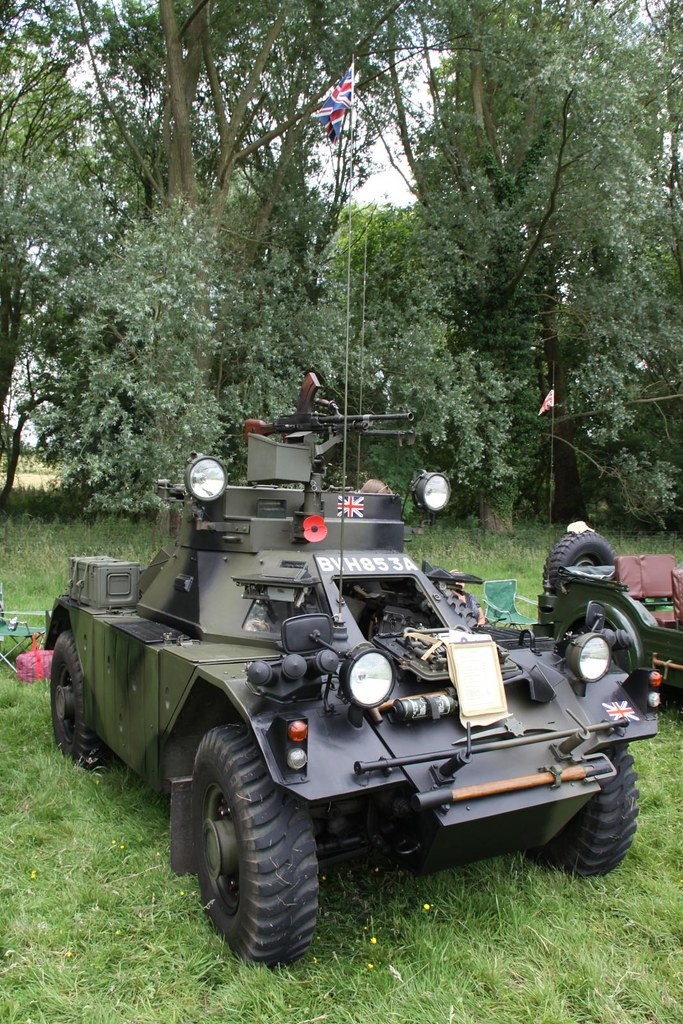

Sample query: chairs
[616,552,677,630]
[482,576,544,632]
[0,580,46,675]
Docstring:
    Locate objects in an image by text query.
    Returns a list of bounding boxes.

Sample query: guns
[244,371,417,473]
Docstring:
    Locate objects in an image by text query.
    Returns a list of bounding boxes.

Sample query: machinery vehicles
[536,520,683,709]
[43,372,668,969]
